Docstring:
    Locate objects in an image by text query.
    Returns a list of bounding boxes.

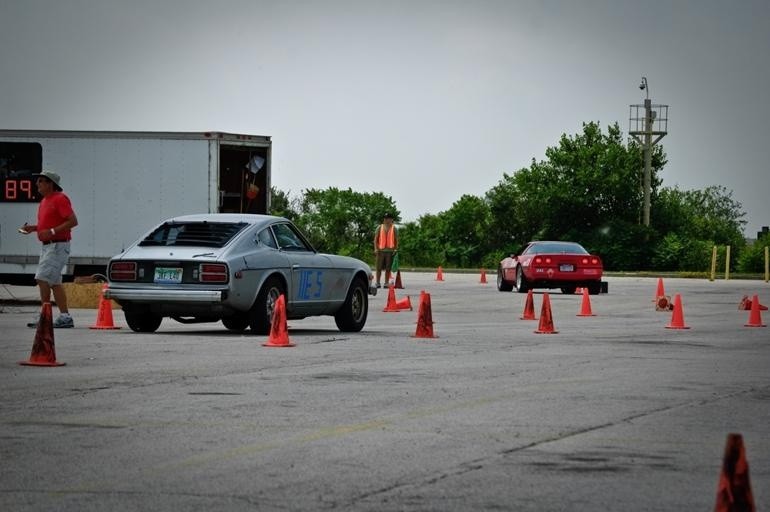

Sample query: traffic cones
[261,294,300,346]
[714,434,756,511]
[384,266,488,338]
[518,288,536,321]
[742,290,768,328]
[86,283,122,329]
[664,293,691,330]
[739,296,769,311]
[19,301,68,366]
[653,278,674,313]
[534,290,560,334]
[576,286,596,317]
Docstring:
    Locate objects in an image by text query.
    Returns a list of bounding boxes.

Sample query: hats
[32,171,62,191]
[384,212,392,218]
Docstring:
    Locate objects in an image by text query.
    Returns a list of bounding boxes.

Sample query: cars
[108,213,373,333]
[495,242,606,294]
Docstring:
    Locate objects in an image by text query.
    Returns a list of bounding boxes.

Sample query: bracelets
[50,228,56,236]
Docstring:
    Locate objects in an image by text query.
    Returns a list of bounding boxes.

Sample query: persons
[372,212,398,288]
[19,171,78,328]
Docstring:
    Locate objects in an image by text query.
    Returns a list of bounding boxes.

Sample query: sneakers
[375,283,381,288]
[27,316,39,327]
[384,283,388,288]
[53,313,74,328]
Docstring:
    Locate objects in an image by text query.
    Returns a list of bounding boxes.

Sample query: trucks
[0,128,271,284]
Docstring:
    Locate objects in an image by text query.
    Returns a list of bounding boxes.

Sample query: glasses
[39,179,42,182]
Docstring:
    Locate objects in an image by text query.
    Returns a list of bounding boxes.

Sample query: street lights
[635,74,653,226]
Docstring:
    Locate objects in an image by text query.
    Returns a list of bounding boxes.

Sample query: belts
[43,239,67,245]
